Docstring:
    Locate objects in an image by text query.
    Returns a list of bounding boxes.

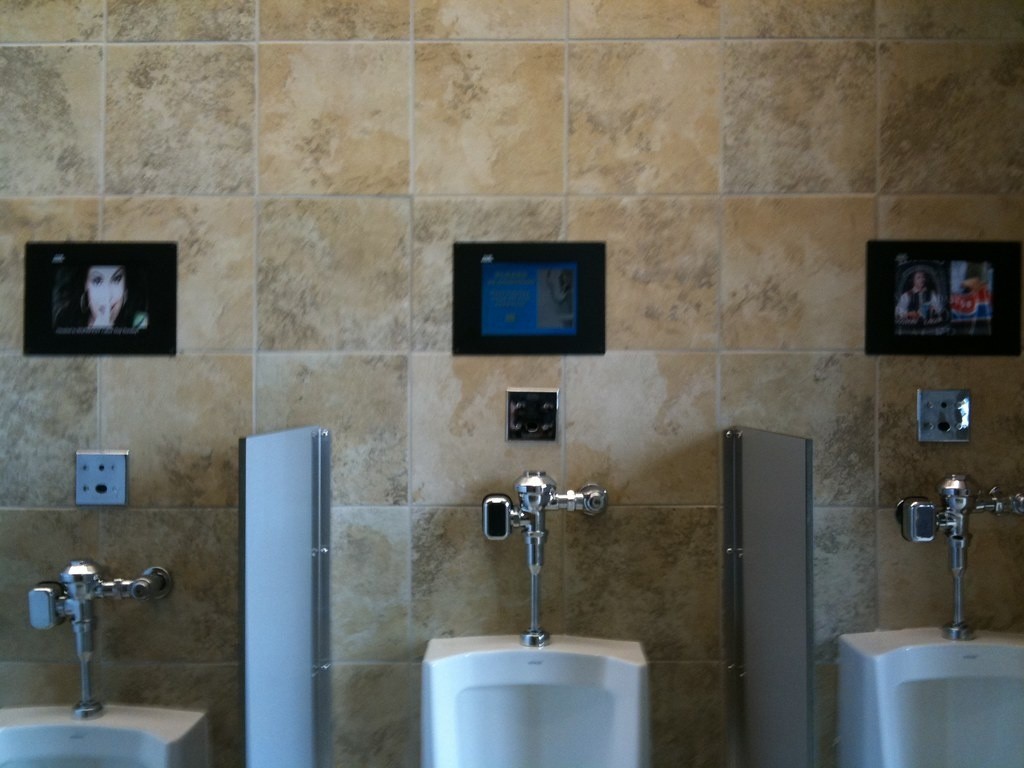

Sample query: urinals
[418,632,653,767]
[0,705,207,768]
[837,626,1024,768]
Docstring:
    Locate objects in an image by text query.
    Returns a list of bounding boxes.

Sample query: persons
[896,269,944,328]
[84,265,147,328]
[961,272,988,294]
